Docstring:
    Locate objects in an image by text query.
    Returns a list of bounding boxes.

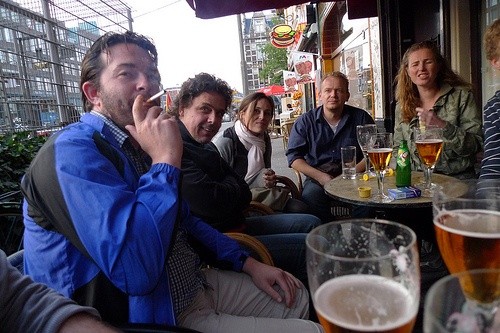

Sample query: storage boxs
[388,186,421,200]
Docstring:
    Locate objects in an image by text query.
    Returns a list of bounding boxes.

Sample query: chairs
[282,122,295,150]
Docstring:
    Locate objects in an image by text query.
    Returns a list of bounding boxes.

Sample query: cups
[358,186,372,198]
[423,267,500,333]
[306,218,422,333]
[341,146,357,180]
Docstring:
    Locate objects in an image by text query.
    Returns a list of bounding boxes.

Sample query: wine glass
[356,125,378,177]
[413,125,444,198]
[365,133,394,203]
[431,177,500,333]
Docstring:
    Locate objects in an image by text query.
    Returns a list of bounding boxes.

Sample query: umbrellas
[259,85,284,120]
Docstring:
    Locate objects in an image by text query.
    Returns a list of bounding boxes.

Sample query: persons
[20,31,324,333]
[0,249,122,333]
[285,71,384,224]
[393,41,482,186]
[475,19,500,211]
[146,73,320,323]
[215,91,309,214]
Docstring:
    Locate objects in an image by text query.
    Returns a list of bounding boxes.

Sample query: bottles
[395,140,412,188]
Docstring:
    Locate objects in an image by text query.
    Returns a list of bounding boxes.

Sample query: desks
[323,169,468,223]
